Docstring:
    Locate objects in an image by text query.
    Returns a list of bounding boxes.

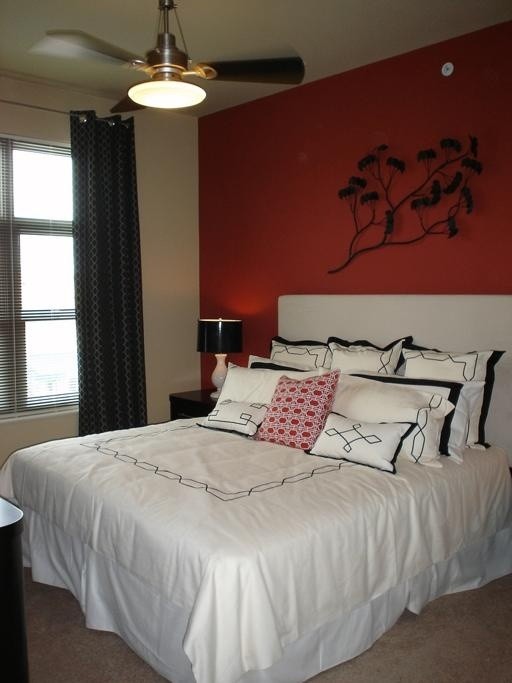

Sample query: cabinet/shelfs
[0,495,30,683]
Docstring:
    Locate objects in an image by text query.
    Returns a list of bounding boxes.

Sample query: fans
[46,1,305,115]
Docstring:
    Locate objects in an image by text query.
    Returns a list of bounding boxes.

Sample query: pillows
[325,336,414,375]
[247,353,312,372]
[198,398,270,440]
[352,368,488,467]
[269,336,332,371]
[331,373,454,470]
[401,342,505,452]
[305,410,418,473]
[219,360,326,402]
[255,369,340,450]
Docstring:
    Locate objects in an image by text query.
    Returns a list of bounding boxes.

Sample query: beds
[0,289,512,683]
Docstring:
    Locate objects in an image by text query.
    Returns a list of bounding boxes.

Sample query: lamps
[197,318,242,398]
[127,73,207,112]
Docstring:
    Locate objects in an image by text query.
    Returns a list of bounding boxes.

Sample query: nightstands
[169,389,217,421]
